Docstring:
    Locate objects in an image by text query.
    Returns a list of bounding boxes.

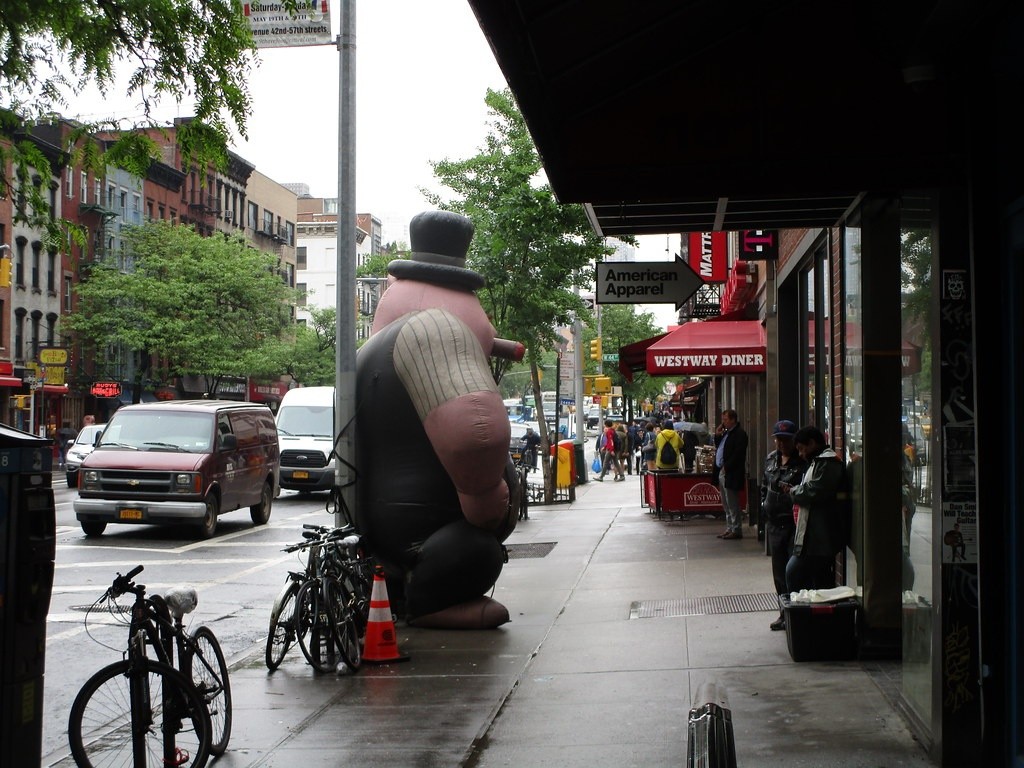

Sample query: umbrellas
[680,422,706,432]
[672,422,689,431]
[633,417,651,424]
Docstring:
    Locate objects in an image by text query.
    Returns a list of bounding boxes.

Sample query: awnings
[809,320,922,378]
[176,374,209,393]
[645,321,766,375]
[141,392,159,403]
[618,309,748,382]
[0,374,22,386]
[118,390,132,403]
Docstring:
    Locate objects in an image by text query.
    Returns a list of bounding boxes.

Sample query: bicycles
[519,441,541,471]
[67,565,232,768]
[264,524,398,673]
[515,464,539,521]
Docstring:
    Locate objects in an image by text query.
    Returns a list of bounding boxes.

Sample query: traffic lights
[17,396,30,410]
[0,258,13,288]
[611,396,623,408]
[590,337,601,361]
[9,397,19,409]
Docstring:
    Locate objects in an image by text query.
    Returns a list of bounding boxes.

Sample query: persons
[582,412,590,441]
[594,411,709,482]
[760,421,849,631]
[520,428,540,472]
[357,209,522,631]
[715,410,749,539]
[57,427,73,467]
[951,523,966,562]
[847,422,916,595]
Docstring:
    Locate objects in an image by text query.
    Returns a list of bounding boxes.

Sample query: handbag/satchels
[593,453,601,473]
[636,449,641,456]
[643,433,654,451]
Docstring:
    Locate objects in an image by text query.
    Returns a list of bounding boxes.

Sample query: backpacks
[661,433,676,464]
[613,429,621,451]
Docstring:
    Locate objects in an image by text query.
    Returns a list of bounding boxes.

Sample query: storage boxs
[778,586,862,663]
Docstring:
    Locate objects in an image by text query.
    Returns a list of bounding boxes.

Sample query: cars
[586,408,607,429]
[602,415,624,425]
[574,406,591,423]
[65,424,123,488]
[824,395,932,466]
[503,391,560,457]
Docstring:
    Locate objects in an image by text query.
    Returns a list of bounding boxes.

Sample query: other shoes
[771,617,784,630]
[717,532,729,538]
[618,477,625,481]
[722,532,742,538]
[596,479,602,481]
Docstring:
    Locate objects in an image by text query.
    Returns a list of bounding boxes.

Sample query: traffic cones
[360,565,410,665]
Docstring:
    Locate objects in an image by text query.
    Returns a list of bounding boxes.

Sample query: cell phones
[722,423,725,428]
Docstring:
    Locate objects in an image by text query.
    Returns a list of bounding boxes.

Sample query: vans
[275,386,336,492]
[72,399,281,538]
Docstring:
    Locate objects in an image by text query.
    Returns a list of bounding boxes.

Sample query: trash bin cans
[573,440,585,483]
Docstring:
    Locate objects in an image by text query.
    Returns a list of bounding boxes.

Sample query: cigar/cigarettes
[490,335,525,360]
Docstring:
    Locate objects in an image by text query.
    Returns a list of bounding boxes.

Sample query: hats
[771,420,797,436]
